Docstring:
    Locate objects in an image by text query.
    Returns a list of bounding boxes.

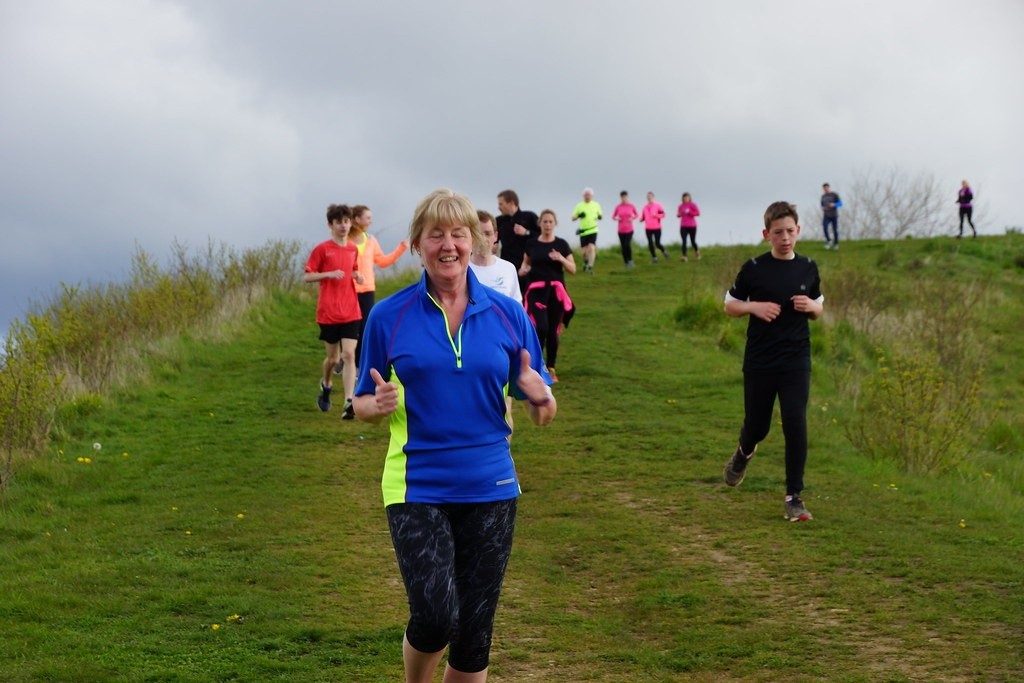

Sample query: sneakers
[341,398,355,419]
[317,377,333,411]
[723,444,758,487]
[783,494,812,521]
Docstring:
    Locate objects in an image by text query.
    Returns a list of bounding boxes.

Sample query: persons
[677,192,702,261]
[469,210,523,312]
[518,210,577,383]
[821,183,843,250]
[639,191,670,263]
[956,180,977,239]
[572,188,602,272]
[333,205,409,380]
[612,190,639,268]
[495,190,541,271]
[724,201,824,521]
[352,187,556,683]
[304,205,364,420]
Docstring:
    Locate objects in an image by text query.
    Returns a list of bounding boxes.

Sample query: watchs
[526,230,530,235]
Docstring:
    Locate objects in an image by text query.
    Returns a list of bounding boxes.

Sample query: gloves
[579,212,585,218]
[598,215,601,220]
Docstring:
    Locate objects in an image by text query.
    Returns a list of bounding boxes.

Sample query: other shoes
[681,256,688,261]
[661,251,669,258]
[695,251,700,256]
[652,257,657,263]
[548,368,559,383]
[625,260,635,270]
[582,261,588,271]
[587,265,593,275]
[334,358,345,374]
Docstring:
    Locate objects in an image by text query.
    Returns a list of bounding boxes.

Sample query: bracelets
[529,399,550,407]
